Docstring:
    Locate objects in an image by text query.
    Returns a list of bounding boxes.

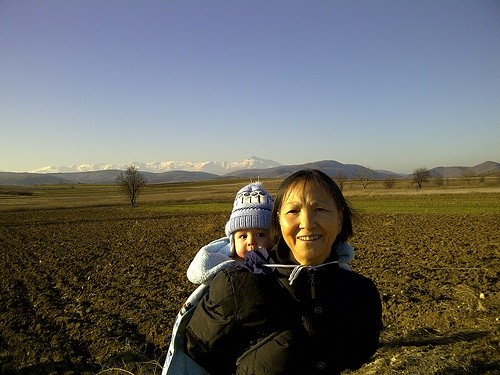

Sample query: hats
[225,182,280,253]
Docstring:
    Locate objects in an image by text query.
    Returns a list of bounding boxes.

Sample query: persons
[162,181,355,375]
[180,168,383,375]
